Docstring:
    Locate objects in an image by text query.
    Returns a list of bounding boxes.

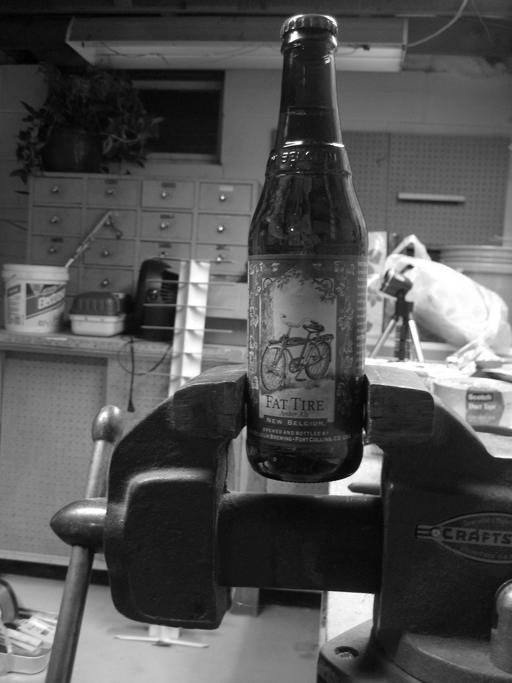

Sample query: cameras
[379,265,413,296]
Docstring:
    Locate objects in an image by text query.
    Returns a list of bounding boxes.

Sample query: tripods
[368,299,428,363]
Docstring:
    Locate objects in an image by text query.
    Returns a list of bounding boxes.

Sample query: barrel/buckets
[441,243,511,323]
[1,262,70,334]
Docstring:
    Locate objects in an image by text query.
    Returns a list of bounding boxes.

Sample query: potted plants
[10,56,165,185]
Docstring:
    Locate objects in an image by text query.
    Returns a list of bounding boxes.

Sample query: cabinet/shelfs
[28,169,258,314]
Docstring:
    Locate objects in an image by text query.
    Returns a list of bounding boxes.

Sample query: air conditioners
[68,15,410,71]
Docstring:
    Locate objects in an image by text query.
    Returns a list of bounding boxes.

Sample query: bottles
[245,10,369,482]
